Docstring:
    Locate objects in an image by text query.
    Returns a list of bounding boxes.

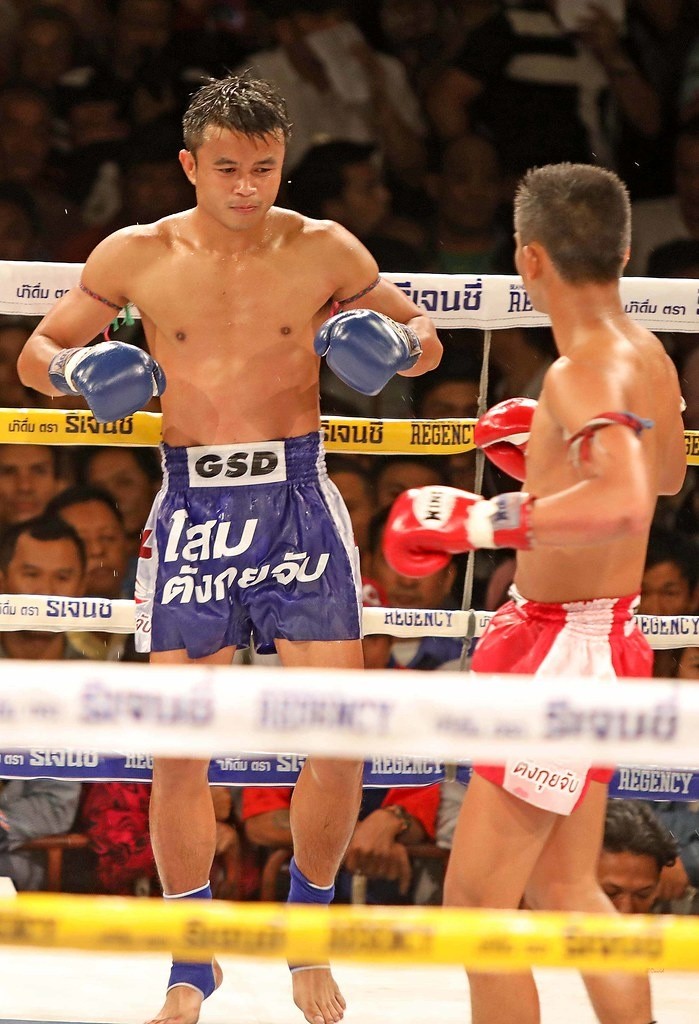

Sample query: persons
[0,0,699,917]
[16,73,443,1024]
[382,165,687,1024]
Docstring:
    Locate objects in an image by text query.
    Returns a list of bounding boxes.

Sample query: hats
[361,575,392,608]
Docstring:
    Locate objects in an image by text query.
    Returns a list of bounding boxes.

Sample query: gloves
[314,308,422,397]
[474,397,539,482]
[48,341,168,424]
[382,485,538,579]
